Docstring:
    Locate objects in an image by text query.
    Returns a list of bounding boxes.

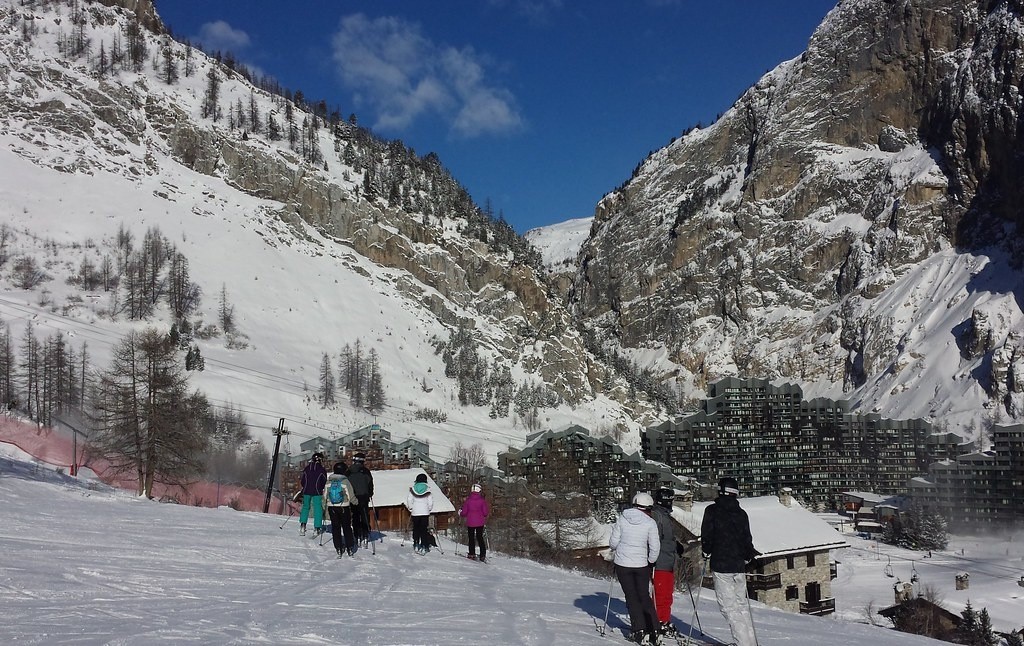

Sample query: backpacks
[330,480,344,504]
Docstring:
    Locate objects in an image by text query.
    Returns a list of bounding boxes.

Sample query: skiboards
[612,610,731,646]
[295,527,325,540]
[459,551,493,566]
[335,538,441,560]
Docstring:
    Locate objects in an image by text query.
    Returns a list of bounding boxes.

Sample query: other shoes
[314,528,321,533]
[301,523,306,532]
[467,555,476,560]
[635,630,661,643]
[415,544,430,554]
[356,533,369,548]
[660,621,677,634]
[347,548,354,556]
[479,556,486,562]
[337,549,342,555]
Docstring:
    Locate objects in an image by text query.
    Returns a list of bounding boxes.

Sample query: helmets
[414,474,427,483]
[470,484,482,493]
[333,462,349,475]
[352,453,366,462]
[655,489,674,509]
[312,453,324,462]
[632,492,654,511]
[716,478,739,497]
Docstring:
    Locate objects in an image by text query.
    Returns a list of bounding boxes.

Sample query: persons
[299,452,326,536]
[457,484,488,562]
[406,474,434,556]
[700,477,758,646]
[649,489,685,638]
[343,453,374,550]
[608,493,663,646]
[321,462,359,560]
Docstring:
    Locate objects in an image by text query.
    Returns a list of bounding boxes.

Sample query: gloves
[676,541,684,555]
[702,552,712,559]
